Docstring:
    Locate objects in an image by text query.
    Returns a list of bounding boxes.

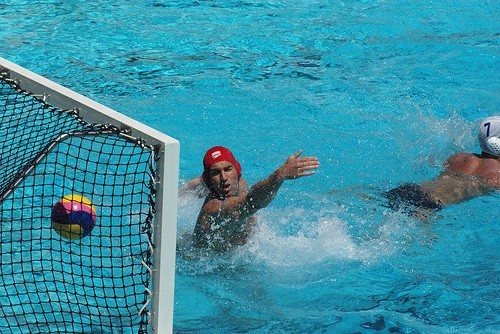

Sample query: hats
[478,117,500,157]
[204,145,239,177]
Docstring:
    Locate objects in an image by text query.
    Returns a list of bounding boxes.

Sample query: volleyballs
[51,195,96,240]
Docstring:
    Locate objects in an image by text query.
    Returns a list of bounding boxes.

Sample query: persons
[176,146,320,281]
[328,116,500,253]
